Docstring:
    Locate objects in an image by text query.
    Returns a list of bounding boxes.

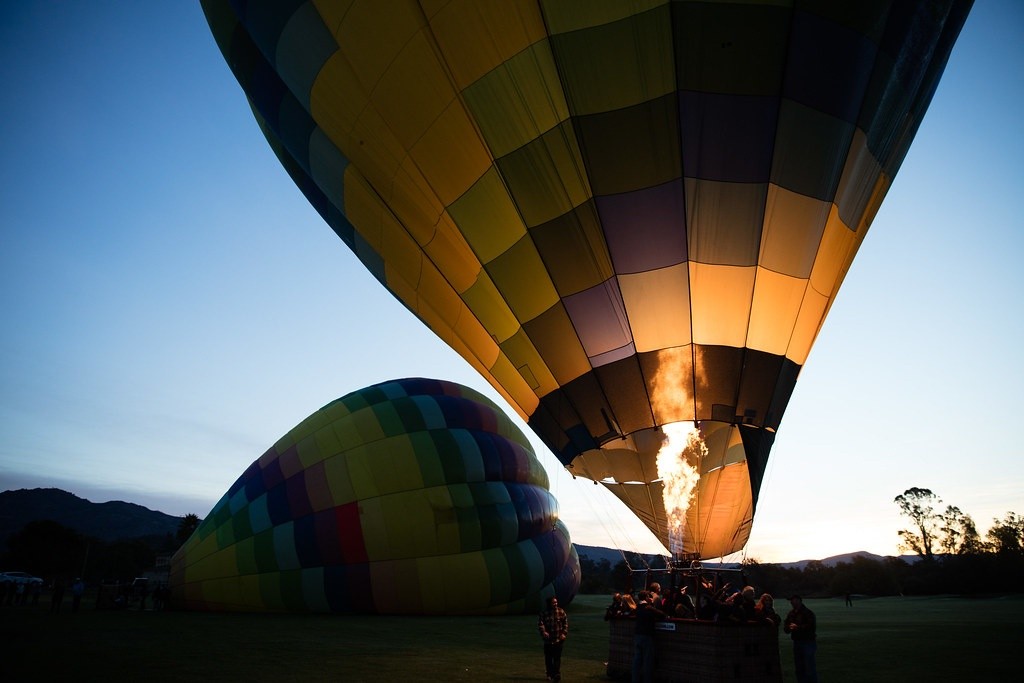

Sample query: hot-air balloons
[195,0,975,683]
[95,377,583,619]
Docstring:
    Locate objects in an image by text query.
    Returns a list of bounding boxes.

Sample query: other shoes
[547,676,551,681]
[553,674,561,683]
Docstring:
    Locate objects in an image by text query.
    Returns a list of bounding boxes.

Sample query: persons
[784,594,818,683]
[845,590,852,607]
[112,575,171,611]
[538,596,568,683]
[603,583,782,683]
[896,593,904,600]
[6,574,84,613]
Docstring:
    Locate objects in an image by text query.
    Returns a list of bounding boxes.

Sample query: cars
[0,571,44,588]
